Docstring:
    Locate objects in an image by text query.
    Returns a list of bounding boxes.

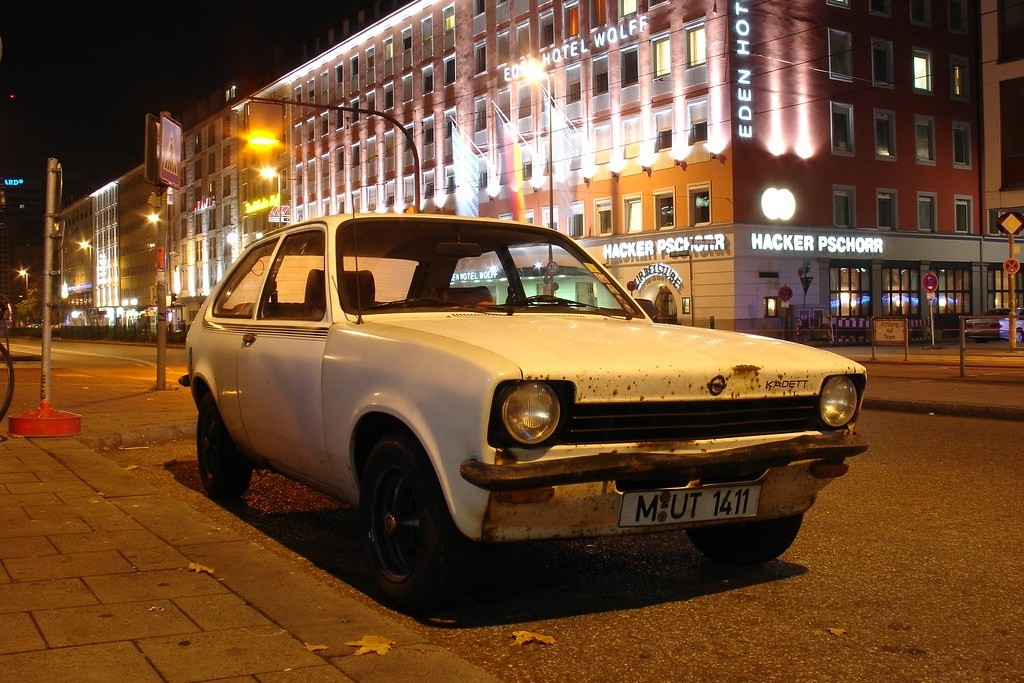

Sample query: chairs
[303,267,375,310]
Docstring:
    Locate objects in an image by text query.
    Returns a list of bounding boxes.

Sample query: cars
[178,212,871,617]
[965,307,1024,344]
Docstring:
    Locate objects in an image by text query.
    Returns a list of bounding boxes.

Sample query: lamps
[674,159,682,166]
[489,195,495,200]
[641,165,649,171]
[435,206,440,211]
[710,152,718,159]
[532,187,540,192]
[583,176,590,183]
[611,171,619,177]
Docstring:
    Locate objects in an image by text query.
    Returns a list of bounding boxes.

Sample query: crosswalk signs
[158,114,182,189]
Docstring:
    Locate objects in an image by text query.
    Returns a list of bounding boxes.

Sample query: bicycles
[0,293,15,421]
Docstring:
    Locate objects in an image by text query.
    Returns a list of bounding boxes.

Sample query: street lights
[20,270,29,300]
[245,95,421,214]
[518,58,554,297]
